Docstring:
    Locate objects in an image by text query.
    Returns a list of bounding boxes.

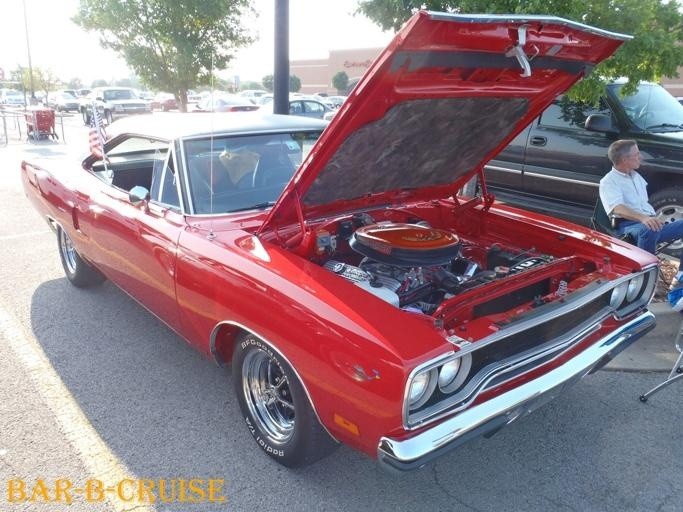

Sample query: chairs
[253,151,296,189]
[589,197,682,287]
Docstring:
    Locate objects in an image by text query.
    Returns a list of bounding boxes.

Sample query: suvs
[465,72,682,266]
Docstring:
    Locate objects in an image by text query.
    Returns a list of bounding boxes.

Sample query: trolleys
[25,110,57,140]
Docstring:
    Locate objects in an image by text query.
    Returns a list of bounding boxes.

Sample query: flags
[89,107,109,160]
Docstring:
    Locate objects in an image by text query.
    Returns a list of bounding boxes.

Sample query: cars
[22,7,662,473]
[0,89,27,107]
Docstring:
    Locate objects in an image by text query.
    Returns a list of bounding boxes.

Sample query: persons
[599,140,683,282]
